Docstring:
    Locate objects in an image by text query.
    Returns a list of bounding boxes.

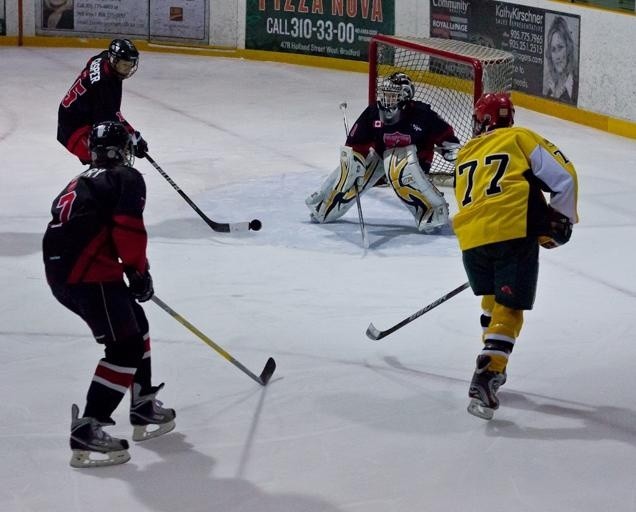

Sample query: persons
[42,119,176,455]
[303,71,461,234]
[42,0,74,29]
[543,16,579,105]
[56,37,149,169]
[449,91,581,412]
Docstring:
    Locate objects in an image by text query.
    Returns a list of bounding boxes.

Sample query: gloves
[538,204,573,249]
[130,131,148,158]
[123,265,153,302]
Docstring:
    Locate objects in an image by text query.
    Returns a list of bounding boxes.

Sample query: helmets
[87,121,135,166]
[471,92,515,135]
[376,72,415,125]
[106,39,139,79]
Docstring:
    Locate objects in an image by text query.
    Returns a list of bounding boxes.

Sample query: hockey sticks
[340,103,370,248]
[151,296,276,386]
[366,283,470,340]
[145,153,251,233]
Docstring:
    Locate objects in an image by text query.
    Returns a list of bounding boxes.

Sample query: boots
[129,381,176,425]
[69,404,130,452]
[469,355,507,409]
